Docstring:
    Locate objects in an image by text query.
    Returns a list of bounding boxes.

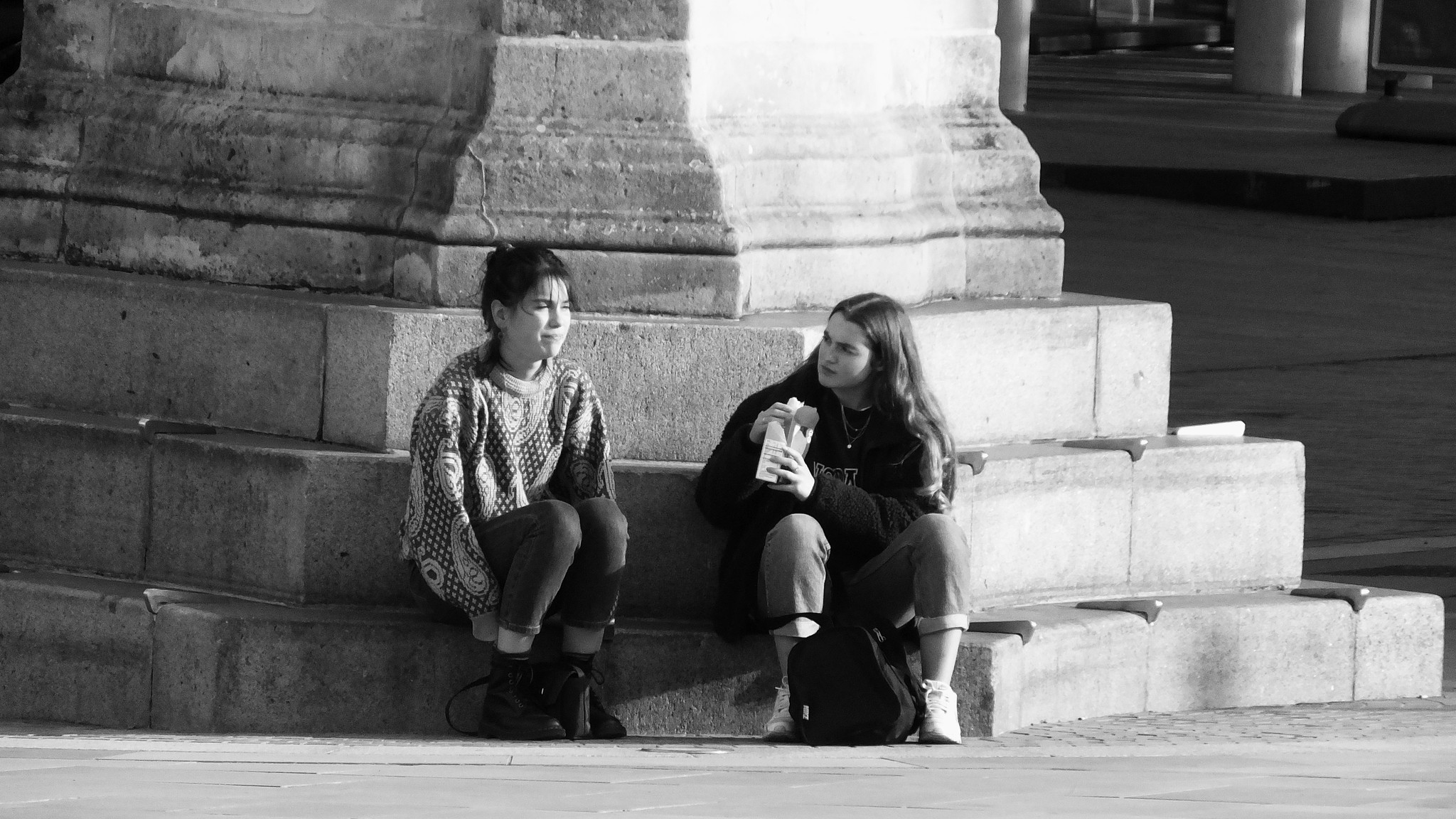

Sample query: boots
[482,659,566,738]
[559,656,627,739]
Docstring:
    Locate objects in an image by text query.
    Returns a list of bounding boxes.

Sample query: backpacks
[765,613,927,745]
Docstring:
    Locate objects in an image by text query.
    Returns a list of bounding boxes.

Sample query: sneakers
[918,679,961,744]
[766,675,796,737]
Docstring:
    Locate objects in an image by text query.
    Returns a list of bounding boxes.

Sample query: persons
[394,242,632,740]
[694,292,972,744]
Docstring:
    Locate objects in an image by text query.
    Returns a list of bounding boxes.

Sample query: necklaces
[841,403,871,448]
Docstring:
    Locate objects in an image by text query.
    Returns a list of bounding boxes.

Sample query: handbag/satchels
[446,661,605,740]
